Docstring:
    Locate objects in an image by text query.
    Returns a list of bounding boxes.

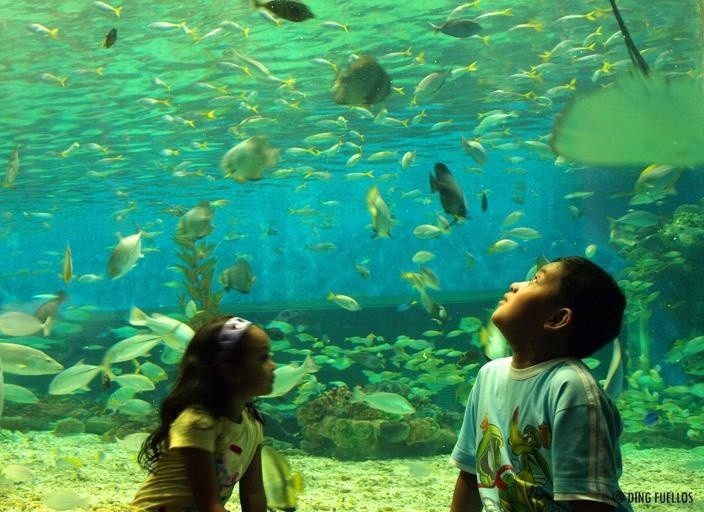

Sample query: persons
[449,256,634,511]
[125,314,277,511]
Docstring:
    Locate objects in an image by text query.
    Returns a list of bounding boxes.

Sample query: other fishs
[0,0,703,511]
